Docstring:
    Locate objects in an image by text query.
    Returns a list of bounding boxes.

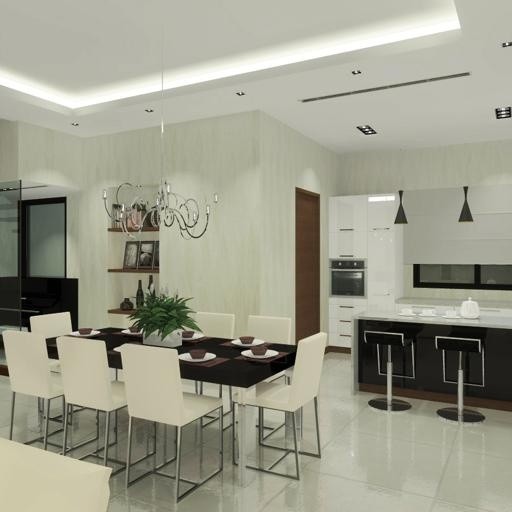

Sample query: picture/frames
[138,240,156,272]
[122,241,141,272]
[153,240,159,273]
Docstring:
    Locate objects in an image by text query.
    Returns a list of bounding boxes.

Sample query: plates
[181,334,205,341]
[241,349,280,359]
[121,328,145,336]
[178,352,217,363]
[68,330,101,336]
[230,337,265,347]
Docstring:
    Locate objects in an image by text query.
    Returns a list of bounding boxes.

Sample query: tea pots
[458,296,480,318]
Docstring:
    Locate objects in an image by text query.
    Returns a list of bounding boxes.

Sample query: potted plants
[126,288,204,348]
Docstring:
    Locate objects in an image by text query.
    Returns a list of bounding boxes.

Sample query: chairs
[433,335,487,424]
[363,330,416,415]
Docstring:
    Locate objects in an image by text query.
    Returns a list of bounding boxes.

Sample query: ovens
[331,269,365,297]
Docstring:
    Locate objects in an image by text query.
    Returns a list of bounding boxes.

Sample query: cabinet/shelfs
[106,228,160,315]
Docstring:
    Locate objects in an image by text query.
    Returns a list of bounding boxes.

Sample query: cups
[80,328,93,334]
[239,336,254,344]
[191,350,205,359]
[183,331,195,337]
[129,326,140,333]
[251,345,267,354]
[398,304,457,321]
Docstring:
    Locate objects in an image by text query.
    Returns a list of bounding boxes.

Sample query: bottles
[136,279,145,309]
[120,297,133,311]
[146,274,157,300]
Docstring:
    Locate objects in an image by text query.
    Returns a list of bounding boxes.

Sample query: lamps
[458,185,473,222]
[101,19,221,241]
[393,190,409,224]
